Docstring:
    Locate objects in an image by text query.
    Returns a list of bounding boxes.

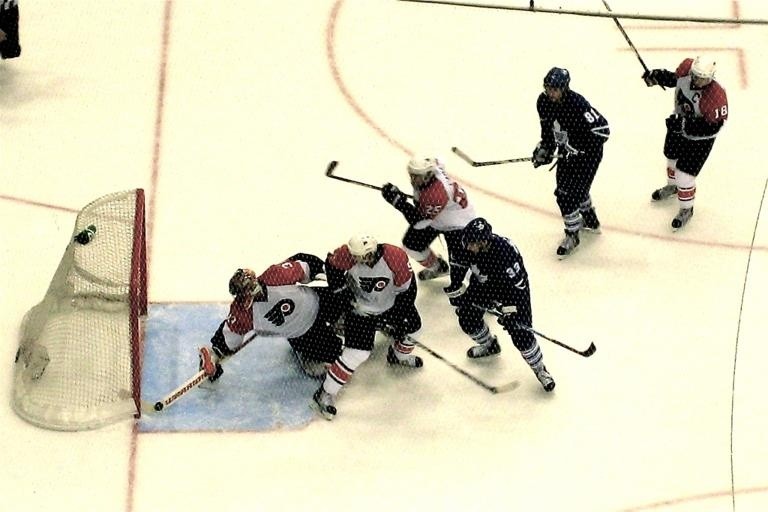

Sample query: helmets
[228,268,257,300]
[460,217,493,251]
[407,154,437,175]
[543,66,571,88]
[691,54,717,81]
[346,233,378,263]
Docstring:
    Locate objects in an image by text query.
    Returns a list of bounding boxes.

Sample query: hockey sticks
[118,329,257,418]
[473,301,595,357]
[452,147,566,167]
[407,335,520,394]
[326,161,414,199]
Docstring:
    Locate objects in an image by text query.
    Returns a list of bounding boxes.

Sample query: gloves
[561,142,579,162]
[643,67,665,88]
[531,141,556,169]
[665,112,686,135]
[197,347,223,383]
[500,304,519,332]
[381,182,406,205]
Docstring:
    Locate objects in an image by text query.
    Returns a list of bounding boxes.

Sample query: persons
[445,217,555,393]
[531,67,611,255]
[312,234,421,420]
[380,157,475,280]
[199,253,346,381]
[642,56,728,228]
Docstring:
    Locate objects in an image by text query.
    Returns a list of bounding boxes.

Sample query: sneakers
[313,383,337,415]
[580,207,601,230]
[467,334,501,358]
[386,342,423,368]
[671,205,694,228]
[556,229,582,255]
[651,183,679,200]
[533,363,555,392]
[417,256,449,281]
